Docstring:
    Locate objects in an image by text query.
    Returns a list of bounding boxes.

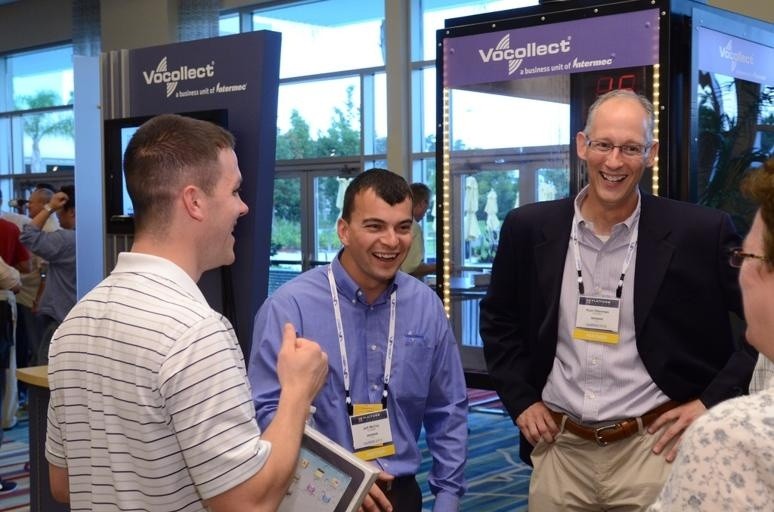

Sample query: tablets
[276,425,380,512]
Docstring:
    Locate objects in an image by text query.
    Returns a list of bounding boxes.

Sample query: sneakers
[1,476,17,495]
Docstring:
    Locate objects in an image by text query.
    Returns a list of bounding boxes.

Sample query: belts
[375,474,416,493]
[549,398,679,448]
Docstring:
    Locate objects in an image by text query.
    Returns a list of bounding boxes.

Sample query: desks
[13,365,95,512]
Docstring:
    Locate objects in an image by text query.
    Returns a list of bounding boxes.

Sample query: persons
[478,87,760,512]
[44,114,329,512]
[1,182,77,496]
[247,168,470,512]
[644,156,774,512]
[400,182,437,279]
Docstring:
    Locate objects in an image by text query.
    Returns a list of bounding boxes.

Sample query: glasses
[586,134,651,156]
[728,246,772,269]
